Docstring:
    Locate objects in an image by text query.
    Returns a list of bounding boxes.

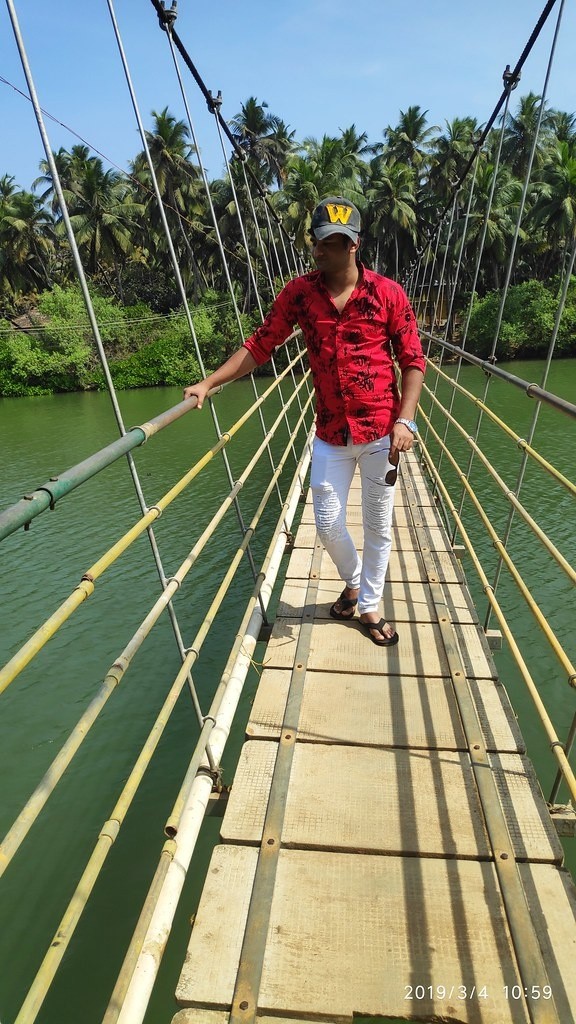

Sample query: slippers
[330,590,357,620]
[359,616,399,646]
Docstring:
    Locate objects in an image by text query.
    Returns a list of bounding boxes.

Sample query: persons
[183,196,426,648]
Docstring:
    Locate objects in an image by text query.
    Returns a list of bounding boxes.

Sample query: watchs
[395,418,418,433]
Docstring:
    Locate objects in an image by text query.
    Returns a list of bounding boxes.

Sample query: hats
[311,196,361,244]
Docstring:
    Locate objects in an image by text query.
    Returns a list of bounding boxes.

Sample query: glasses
[366,448,399,487]
